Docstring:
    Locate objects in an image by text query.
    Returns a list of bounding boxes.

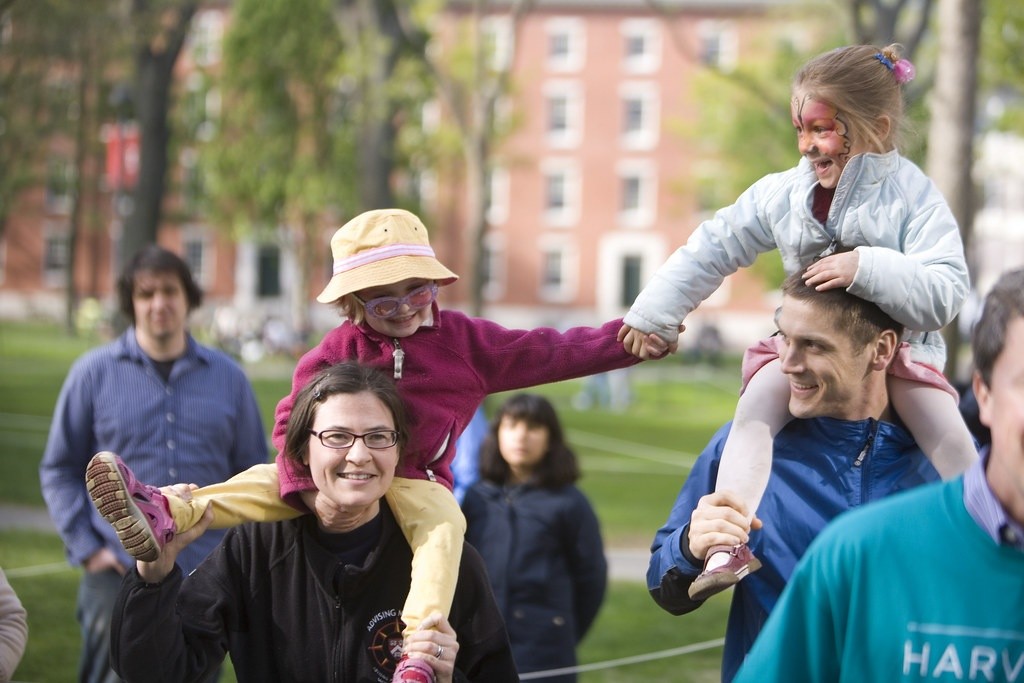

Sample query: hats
[315,209,460,303]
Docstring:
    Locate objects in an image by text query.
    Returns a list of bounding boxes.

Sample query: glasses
[350,278,437,319]
[306,427,401,448]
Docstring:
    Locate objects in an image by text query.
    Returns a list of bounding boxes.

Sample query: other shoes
[392,659,441,683]
[86,451,174,562]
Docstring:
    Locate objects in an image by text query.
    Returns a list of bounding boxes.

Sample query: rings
[434,644,444,660]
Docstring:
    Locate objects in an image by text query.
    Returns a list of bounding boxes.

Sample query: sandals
[688,547,764,599]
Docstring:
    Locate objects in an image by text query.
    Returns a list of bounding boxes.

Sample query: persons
[450,400,496,506]
[457,391,609,683]
[618,45,984,603]
[644,260,994,681]
[109,359,522,683]
[724,264,1024,683]
[84,208,689,683]
[0,568,31,681]
[37,246,270,683]
